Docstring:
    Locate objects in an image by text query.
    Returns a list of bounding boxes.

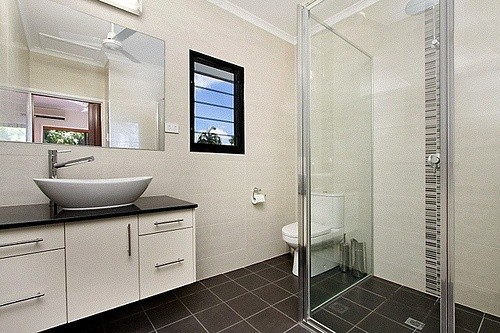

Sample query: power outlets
[165,122,180,134]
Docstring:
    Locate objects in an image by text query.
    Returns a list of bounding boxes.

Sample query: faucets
[47,148,95,177]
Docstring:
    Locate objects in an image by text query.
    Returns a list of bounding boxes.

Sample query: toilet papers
[251,187,267,205]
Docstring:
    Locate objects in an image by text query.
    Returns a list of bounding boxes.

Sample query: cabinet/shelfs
[65,215,139,323]
[0,224,68,333]
[139,207,196,301]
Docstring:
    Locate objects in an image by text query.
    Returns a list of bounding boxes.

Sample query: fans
[38,22,143,64]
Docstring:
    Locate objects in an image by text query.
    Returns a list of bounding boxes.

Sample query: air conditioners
[24,106,66,120]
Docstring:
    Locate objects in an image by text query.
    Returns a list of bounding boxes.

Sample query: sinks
[32,177,151,210]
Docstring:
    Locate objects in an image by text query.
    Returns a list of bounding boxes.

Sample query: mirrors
[0,0,165,152]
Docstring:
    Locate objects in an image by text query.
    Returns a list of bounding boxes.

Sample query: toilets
[282,190,344,277]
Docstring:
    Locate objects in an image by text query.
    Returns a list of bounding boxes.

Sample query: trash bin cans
[350,238,366,278]
